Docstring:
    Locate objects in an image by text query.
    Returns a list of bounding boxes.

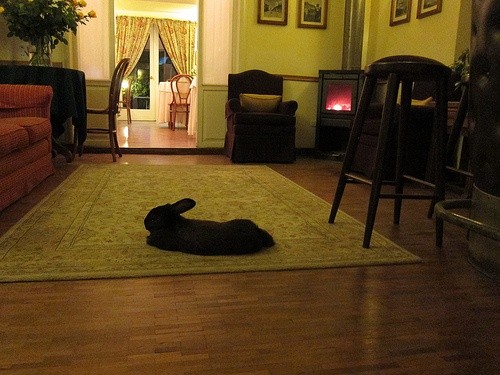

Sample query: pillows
[397,97,432,107]
[239,93,282,112]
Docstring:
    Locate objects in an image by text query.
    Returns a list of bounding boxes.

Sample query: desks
[0,65,88,162]
[426,101,471,188]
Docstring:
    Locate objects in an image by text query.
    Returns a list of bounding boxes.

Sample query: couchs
[349,100,434,181]
[348,72,463,179]
[0,84,53,211]
[224,70,298,164]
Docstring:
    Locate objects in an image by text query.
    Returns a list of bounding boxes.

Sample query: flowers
[0,0,97,66]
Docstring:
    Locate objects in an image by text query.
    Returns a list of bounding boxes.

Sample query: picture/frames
[256,0,288,26]
[390,0,412,26]
[297,0,329,29]
[416,0,442,19]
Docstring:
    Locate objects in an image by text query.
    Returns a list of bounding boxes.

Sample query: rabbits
[144,197,277,256]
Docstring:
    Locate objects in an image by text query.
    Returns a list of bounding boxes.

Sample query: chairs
[72,58,130,162]
[168,75,194,131]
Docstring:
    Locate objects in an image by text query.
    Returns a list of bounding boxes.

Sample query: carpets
[0,165,424,284]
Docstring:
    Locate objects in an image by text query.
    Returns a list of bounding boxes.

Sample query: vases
[22,36,53,67]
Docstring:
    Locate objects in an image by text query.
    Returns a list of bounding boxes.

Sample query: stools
[328,56,452,249]
